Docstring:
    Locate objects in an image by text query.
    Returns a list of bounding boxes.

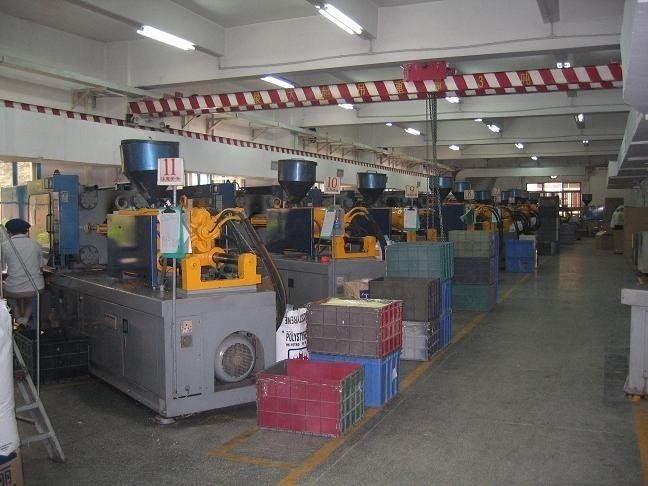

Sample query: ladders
[12,340,66,462]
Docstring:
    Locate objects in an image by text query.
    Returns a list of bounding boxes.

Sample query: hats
[4,218,32,233]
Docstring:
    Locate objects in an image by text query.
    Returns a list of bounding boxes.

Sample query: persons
[610,205,624,230]
[1,218,45,333]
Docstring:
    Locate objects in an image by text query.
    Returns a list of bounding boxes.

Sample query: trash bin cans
[621,288,648,396]
